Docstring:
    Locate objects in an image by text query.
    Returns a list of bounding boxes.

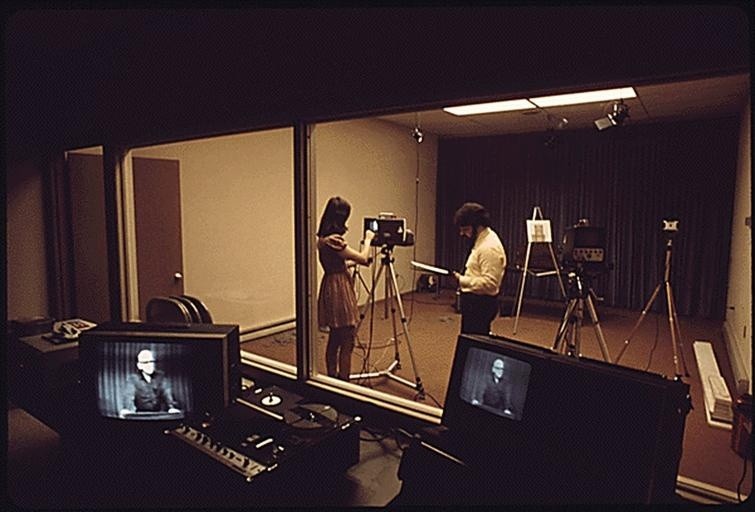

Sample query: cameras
[361,211,415,249]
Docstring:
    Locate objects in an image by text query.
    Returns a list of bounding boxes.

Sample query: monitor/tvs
[77,321,240,423]
[458,345,533,422]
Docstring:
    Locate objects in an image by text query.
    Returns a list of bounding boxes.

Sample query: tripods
[614,242,690,379]
[334,248,425,399]
[552,294,613,364]
[511,207,567,336]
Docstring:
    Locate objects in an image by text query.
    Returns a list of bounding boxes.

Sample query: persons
[452,203,507,334]
[472,358,513,415]
[125,349,173,413]
[318,197,370,383]
